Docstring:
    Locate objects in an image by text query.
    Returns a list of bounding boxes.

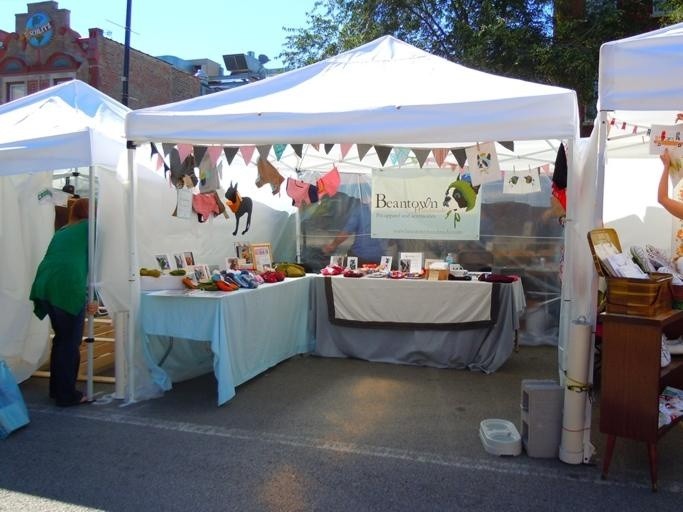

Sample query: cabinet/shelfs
[598,311,683,492]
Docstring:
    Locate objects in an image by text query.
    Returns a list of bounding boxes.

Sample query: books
[599,251,649,280]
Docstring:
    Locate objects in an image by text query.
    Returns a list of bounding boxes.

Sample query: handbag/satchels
[275,263,305,277]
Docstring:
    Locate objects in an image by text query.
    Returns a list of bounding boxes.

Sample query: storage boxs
[585,228,674,316]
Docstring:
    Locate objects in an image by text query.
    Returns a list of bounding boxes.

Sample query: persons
[320,195,390,268]
[28,197,102,406]
[657,147,683,225]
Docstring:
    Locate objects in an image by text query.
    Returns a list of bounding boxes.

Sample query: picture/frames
[249,243,273,272]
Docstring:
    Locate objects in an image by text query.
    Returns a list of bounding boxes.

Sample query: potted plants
[139,268,186,290]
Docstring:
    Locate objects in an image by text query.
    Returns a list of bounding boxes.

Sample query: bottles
[446,252,453,264]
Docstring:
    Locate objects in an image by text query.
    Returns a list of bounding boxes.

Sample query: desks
[312,275,527,375]
[138,272,314,406]
